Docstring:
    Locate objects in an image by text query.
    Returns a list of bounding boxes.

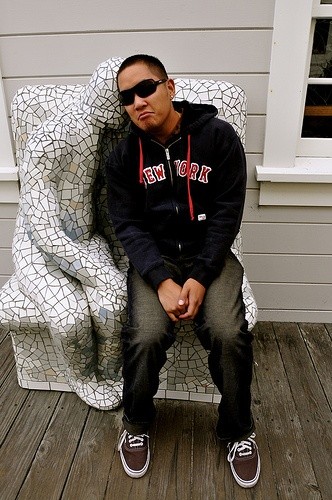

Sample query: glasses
[118,78,167,106]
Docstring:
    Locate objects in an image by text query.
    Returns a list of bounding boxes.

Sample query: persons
[11,57,123,411]
[104,53,262,489]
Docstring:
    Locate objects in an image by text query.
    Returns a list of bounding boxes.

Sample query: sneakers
[120,425,151,478]
[227,433,260,488]
